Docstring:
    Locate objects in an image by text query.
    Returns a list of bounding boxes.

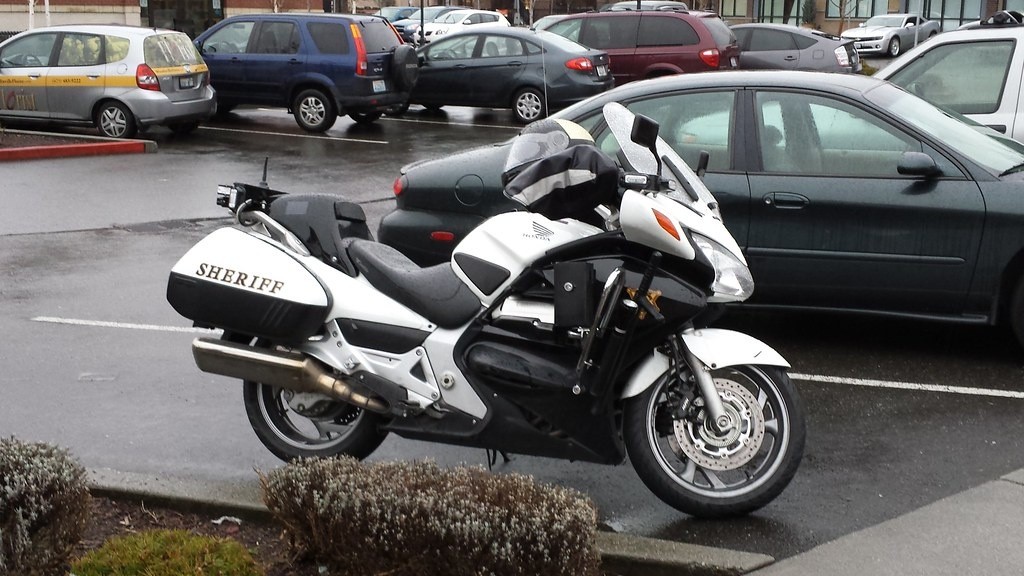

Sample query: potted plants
[801,0,818,29]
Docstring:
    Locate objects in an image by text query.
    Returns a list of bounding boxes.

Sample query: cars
[673,24,1024,165]
[376,68,1024,359]
[371,6,511,58]
[602,1,690,13]
[726,22,864,73]
[840,13,941,58]
[382,26,615,124]
[0,23,218,140]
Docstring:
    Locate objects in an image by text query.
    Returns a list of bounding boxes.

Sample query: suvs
[516,9,741,87]
[192,11,420,133]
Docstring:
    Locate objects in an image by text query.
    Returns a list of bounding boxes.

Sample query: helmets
[501,120,597,187]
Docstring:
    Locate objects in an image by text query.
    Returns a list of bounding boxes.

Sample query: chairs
[263,31,277,53]
[506,40,518,55]
[486,42,499,56]
[763,126,793,172]
[583,26,598,48]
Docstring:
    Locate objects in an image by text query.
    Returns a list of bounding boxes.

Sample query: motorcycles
[162,102,806,522]
